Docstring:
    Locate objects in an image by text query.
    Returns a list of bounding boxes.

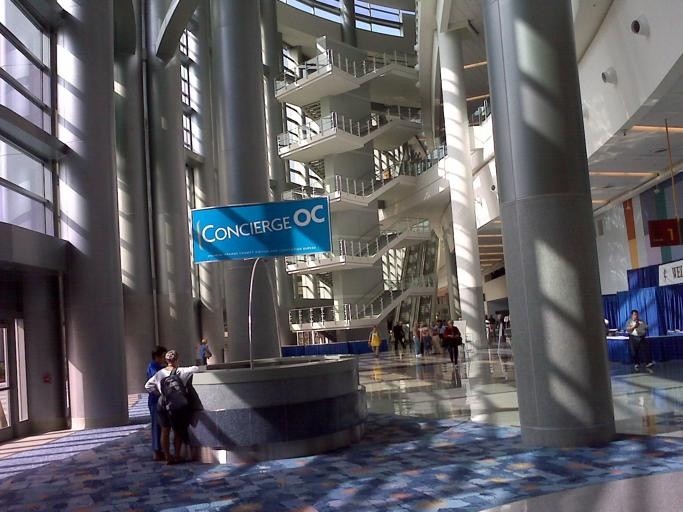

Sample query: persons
[485,314,507,344]
[195,339,211,367]
[441,320,461,368]
[624,310,656,370]
[392,319,463,359]
[144,350,199,464]
[145,345,170,461]
[368,325,382,358]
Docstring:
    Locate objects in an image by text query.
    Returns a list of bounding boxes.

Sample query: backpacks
[160,367,190,420]
